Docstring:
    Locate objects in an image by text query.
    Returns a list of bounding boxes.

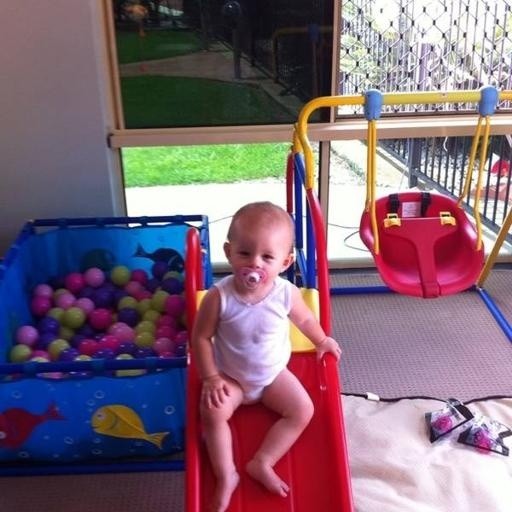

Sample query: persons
[191,201,341,512]
